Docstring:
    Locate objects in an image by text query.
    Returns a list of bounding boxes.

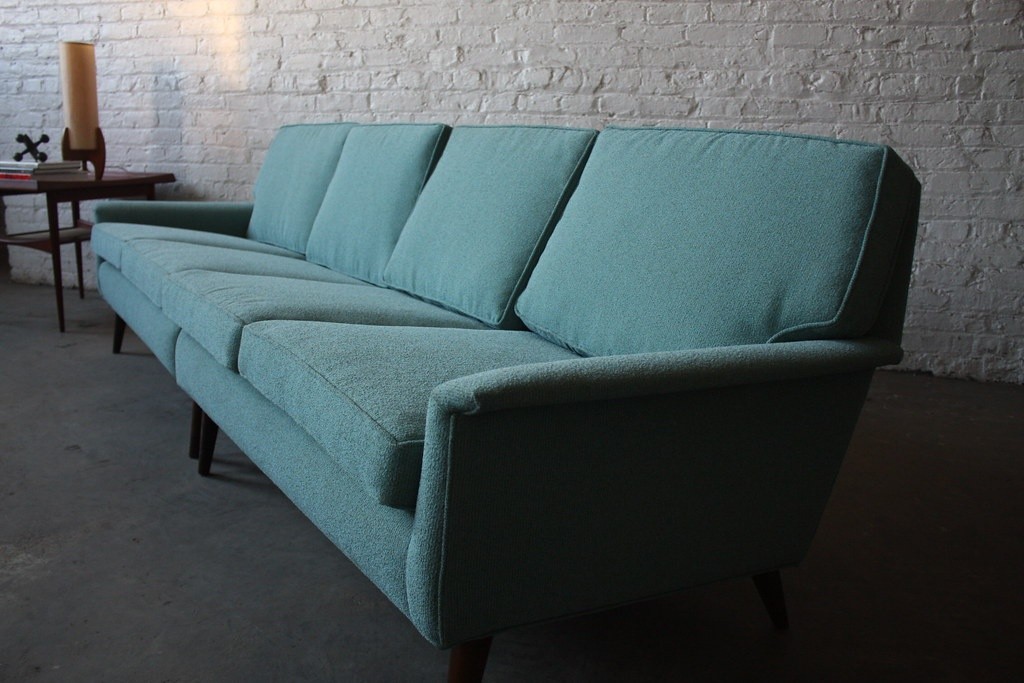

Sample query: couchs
[91,121,924,683]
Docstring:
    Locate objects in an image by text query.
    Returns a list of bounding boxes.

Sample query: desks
[0,171,177,333]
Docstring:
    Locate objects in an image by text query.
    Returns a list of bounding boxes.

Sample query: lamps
[60,39,107,181]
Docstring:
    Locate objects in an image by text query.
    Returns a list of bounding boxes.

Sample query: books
[0,160,91,180]
